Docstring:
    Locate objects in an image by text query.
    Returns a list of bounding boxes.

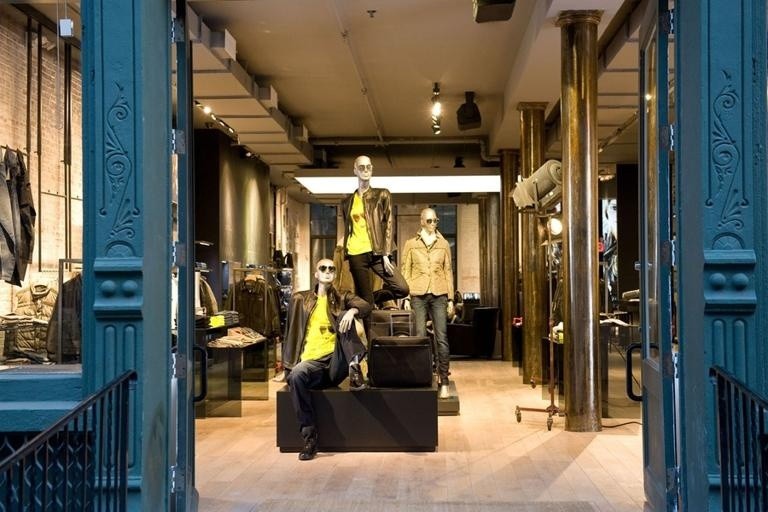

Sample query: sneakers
[440,385,450,398]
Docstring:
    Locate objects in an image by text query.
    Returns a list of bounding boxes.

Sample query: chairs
[446,308,502,359]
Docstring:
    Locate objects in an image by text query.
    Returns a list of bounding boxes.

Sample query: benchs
[277,376,439,453]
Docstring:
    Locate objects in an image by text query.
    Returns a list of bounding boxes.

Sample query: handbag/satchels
[369,336,433,387]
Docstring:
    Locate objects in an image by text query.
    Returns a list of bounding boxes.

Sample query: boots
[299,426,319,460]
[349,364,367,392]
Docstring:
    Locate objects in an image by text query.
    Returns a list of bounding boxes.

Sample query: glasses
[426,218,440,225]
[358,164,372,171]
[318,265,336,273]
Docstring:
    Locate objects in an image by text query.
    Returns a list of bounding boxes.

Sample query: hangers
[244,268,265,283]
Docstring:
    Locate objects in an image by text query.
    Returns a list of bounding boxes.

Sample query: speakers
[472,0,516,23]
[456,102,482,131]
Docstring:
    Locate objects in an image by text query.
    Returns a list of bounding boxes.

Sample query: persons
[270,258,374,460]
[334,154,412,345]
[397,206,456,401]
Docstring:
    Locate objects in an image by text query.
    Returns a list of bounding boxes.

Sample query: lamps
[429,82,443,135]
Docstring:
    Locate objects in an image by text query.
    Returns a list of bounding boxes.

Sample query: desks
[195,320,240,402]
